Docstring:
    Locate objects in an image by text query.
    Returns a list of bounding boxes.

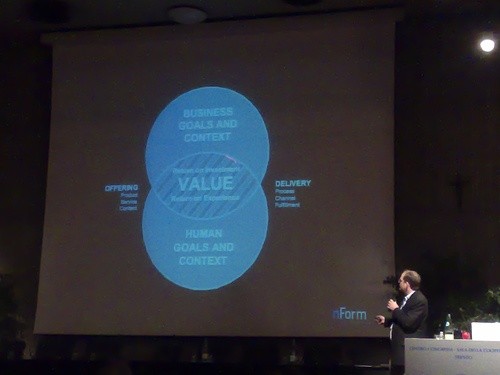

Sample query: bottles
[443,314,456,342]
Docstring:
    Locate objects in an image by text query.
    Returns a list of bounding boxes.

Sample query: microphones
[388,290,398,311]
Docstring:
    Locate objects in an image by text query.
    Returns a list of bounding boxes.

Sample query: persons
[375,270,430,375]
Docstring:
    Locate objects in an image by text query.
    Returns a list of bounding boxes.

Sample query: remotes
[374,316,380,321]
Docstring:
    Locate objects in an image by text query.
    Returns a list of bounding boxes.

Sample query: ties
[389,299,407,341]
